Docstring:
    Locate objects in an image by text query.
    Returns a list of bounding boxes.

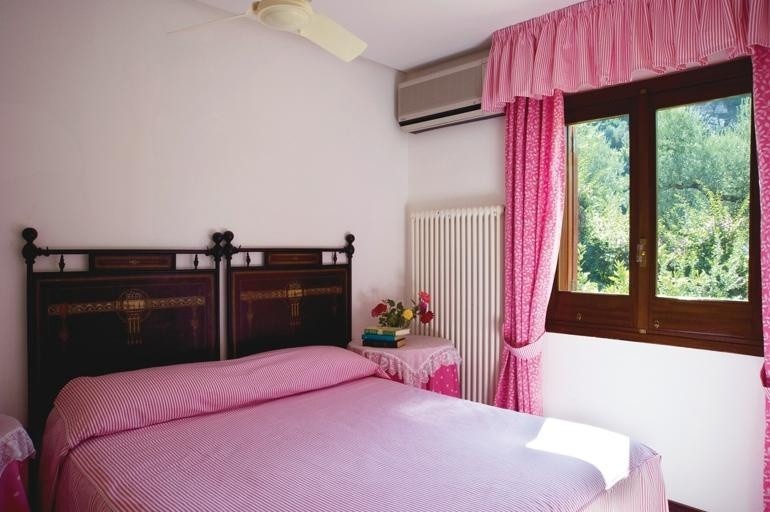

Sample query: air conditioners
[393,53,507,137]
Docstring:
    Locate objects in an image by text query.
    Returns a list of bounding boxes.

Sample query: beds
[16,228,664,511]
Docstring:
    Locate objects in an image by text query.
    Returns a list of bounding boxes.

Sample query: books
[362,338,408,348]
[361,334,405,341]
[364,326,410,336]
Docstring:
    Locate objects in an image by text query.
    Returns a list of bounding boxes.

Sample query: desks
[345,335,461,401]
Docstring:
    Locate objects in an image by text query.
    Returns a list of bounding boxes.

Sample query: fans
[168,1,369,65]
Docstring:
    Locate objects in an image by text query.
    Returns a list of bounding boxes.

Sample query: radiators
[409,204,505,406]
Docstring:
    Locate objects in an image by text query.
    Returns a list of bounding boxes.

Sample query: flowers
[370,292,436,330]
